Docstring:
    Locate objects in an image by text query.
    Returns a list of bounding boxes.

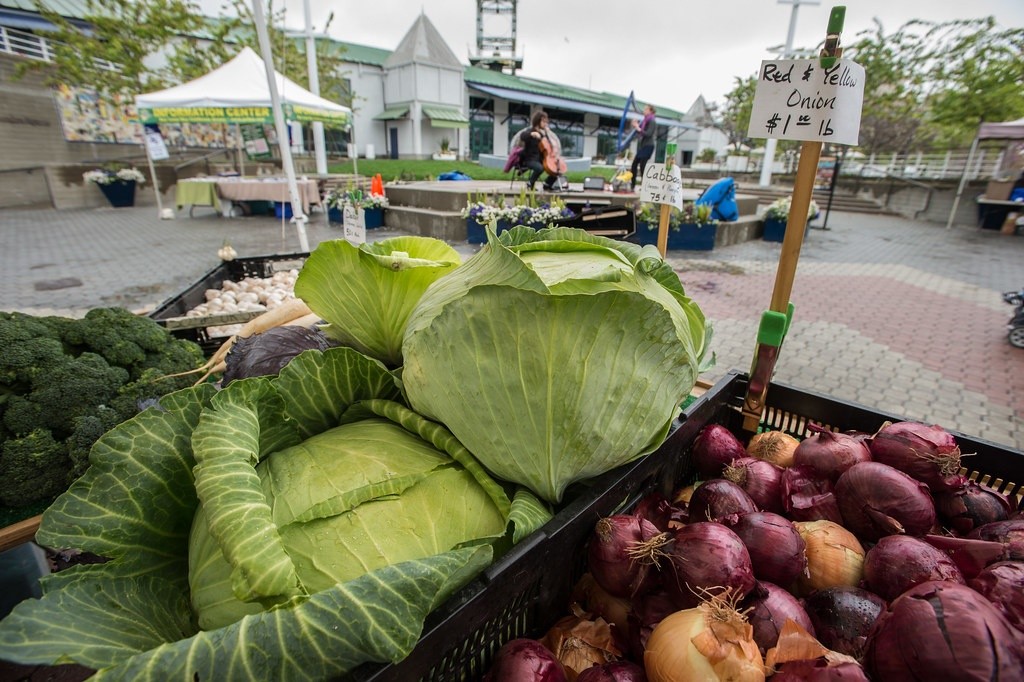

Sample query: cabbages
[294,238,458,362]
[403,224,713,507]
[1,349,553,681]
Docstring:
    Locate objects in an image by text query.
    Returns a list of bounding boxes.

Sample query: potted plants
[631,203,717,252]
[85,160,145,210]
[761,194,820,243]
[461,189,575,244]
[327,188,391,228]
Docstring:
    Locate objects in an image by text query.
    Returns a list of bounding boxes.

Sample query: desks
[173,175,324,226]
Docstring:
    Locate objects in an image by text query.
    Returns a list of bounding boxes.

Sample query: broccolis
[0,308,208,529]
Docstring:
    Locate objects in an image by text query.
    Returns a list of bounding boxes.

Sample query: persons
[630,105,656,194]
[520,111,557,191]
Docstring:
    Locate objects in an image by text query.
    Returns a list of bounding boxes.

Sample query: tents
[944,114,1023,239]
[133,46,362,248]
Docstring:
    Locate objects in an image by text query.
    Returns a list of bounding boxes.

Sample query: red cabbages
[220,325,341,387]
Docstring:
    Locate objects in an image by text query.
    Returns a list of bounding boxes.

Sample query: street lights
[761,0,819,188]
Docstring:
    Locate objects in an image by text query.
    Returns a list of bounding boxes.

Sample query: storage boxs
[0,238,1024,682]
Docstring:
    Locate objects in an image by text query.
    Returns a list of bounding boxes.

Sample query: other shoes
[527,182,538,191]
[543,181,554,191]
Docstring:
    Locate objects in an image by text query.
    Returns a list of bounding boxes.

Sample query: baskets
[137,246,312,362]
[349,368,1023,681]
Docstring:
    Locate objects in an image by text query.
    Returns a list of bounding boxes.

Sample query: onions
[501,417,1024,682]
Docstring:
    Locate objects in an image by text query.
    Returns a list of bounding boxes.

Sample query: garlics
[182,242,300,318]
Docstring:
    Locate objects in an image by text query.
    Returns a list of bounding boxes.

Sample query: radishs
[153,300,330,387]
